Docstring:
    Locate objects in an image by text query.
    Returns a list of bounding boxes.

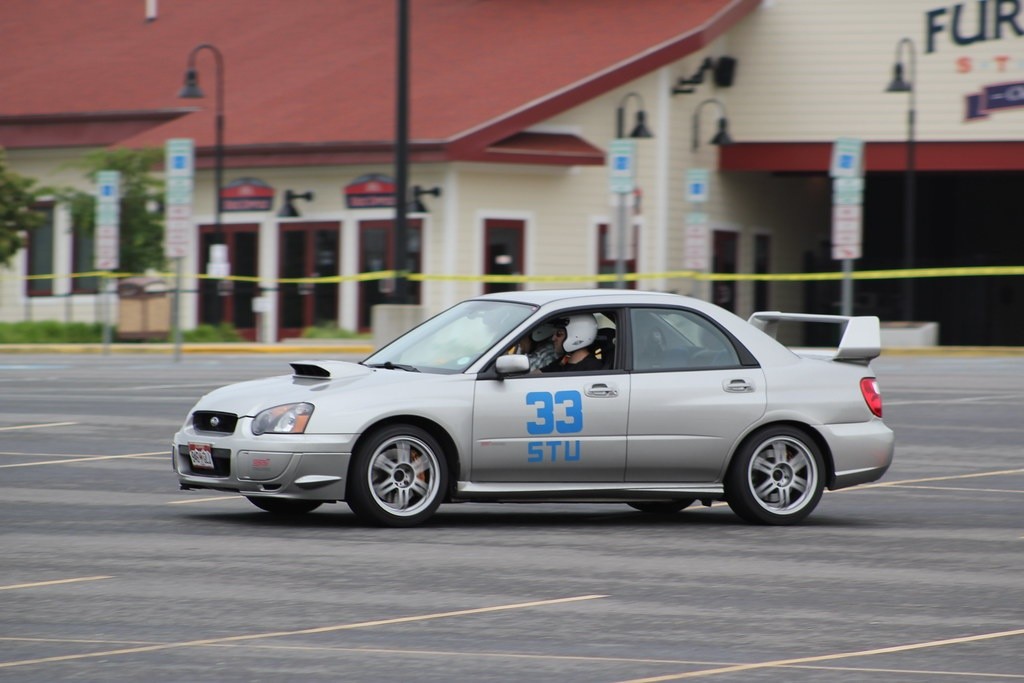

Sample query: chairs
[588,327,616,369]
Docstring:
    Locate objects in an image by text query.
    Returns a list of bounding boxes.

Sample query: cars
[172,288,894,529]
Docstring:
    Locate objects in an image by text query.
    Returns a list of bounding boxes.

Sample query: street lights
[179,42,221,326]
[614,88,657,283]
[882,37,917,324]
[689,95,732,296]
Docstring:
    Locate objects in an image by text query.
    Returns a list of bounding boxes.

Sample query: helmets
[560,313,598,353]
[532,323,556,342]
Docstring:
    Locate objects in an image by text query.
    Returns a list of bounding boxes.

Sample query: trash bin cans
[113,276,170,341]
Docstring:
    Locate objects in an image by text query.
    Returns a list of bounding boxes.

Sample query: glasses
[551,330,566,338]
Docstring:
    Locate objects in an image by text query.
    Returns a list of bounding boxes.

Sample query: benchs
[638,344,734,371]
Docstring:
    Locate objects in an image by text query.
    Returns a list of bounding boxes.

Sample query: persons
[519,312,603,374]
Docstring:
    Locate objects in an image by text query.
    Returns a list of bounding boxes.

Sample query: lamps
[406,184,441,215]
[675,54,736,92]
[276,189,314,216]
